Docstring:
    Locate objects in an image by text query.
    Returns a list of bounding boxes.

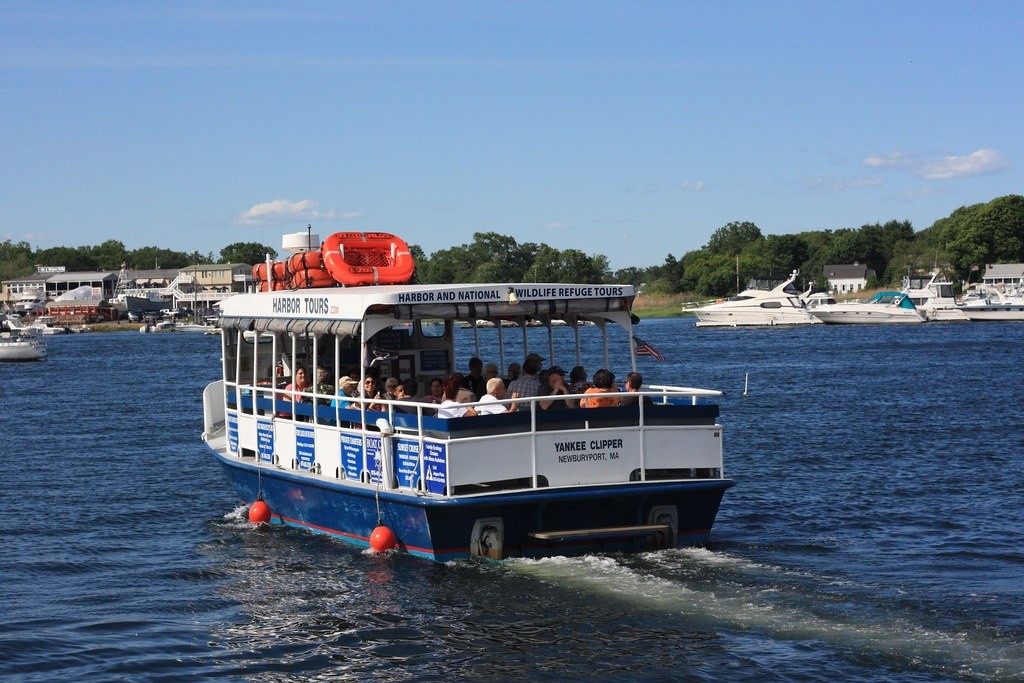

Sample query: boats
[727,281,837,306]
[808,290,928,324]
[900,267,1024,323]
[680,268,822,327]
[252,250,333,293]
[319,230,417,285]
[0,291,66,361]
[201,279,738,565]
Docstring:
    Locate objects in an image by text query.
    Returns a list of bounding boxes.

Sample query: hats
[339,375,358,387]
[548,366,569,376]
[386,377,398,387]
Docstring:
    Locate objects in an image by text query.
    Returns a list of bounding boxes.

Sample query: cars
[127,307,193,323]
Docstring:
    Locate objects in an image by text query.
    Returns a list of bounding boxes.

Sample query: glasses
[365,380,376,385]
[555,372,566,377]
[624,380,629,385]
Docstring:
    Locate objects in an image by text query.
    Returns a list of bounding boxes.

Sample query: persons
[429,354,575,417]
[580,369,623,409]
[620,372,652,407]
[279,365,435,430]
[569,366,592,403]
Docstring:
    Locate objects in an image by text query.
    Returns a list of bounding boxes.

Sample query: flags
[634,336,665,363]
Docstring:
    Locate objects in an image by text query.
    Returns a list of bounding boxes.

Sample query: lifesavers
[715,299,722,303]
[251,252,340,293]
[321,231,414,284]
[894,300,900,304]
